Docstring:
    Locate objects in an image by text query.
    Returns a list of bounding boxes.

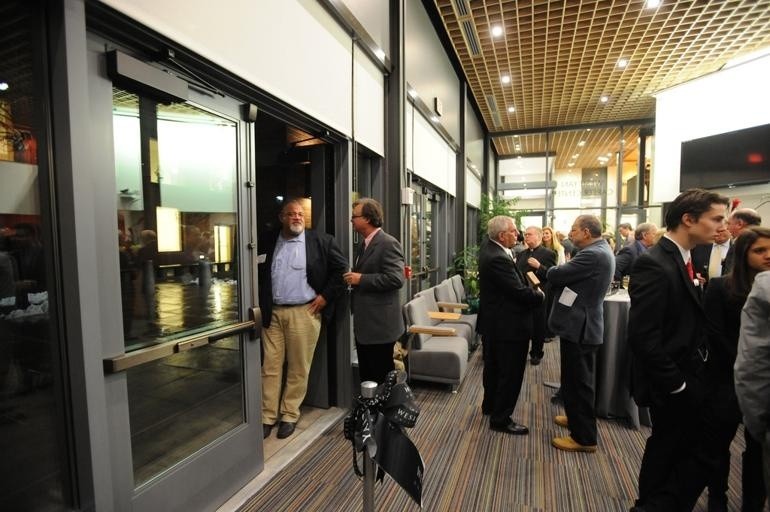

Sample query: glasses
[351,215,364,220]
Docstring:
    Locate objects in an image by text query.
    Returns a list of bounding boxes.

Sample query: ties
[685,256,694,282]
[510,249,519,268]
[528,249,535,258]
[356,239,366,267]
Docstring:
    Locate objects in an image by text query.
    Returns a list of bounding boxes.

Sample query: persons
[259,198,349,442]
[136,230,158,321]
[342,198,404,386]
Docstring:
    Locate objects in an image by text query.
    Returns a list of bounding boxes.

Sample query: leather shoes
[551,435,597,454]
[277,420,297,439]
[530,355,541,365]
[554,415,570,428]
[263,421,276,440]
[544,337,551,343]
[489,421,528,435]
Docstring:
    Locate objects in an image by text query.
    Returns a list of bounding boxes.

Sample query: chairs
[452,274,469,303]
[435,278,477,332]
[403,295,469,392]
[414,288,472,349]
